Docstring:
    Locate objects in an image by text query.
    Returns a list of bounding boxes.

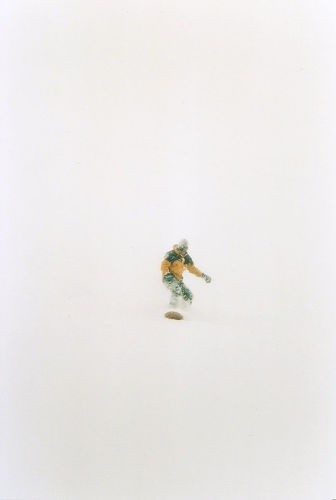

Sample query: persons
[160,237,211,320]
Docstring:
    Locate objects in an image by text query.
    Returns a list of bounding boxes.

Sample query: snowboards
[165,311,182,320]
